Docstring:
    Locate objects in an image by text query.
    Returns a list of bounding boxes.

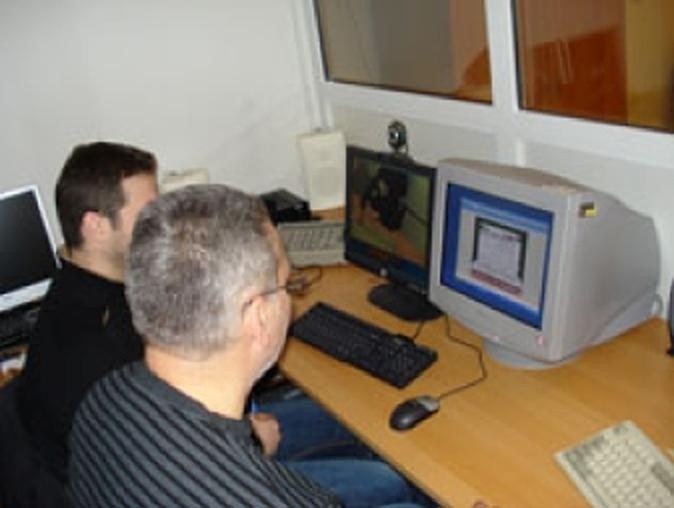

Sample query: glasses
[241,273,302,319]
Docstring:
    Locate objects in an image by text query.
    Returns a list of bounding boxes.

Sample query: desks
[276,206,674,506]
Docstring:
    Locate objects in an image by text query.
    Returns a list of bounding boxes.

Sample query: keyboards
[290,301,437,389]
[551,421,674,508]
[0,307,47,349]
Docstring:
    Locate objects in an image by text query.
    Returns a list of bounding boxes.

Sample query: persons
[19,144,374,508]
[60,185,431,508]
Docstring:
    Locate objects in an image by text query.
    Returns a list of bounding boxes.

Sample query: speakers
[300,134,346,213]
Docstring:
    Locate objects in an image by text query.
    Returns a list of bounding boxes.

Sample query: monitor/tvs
[342,147,445,321]
[0,183,67,315]
[427,160,662,370]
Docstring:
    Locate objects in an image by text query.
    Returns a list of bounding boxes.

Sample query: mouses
[387,395,440,428]
